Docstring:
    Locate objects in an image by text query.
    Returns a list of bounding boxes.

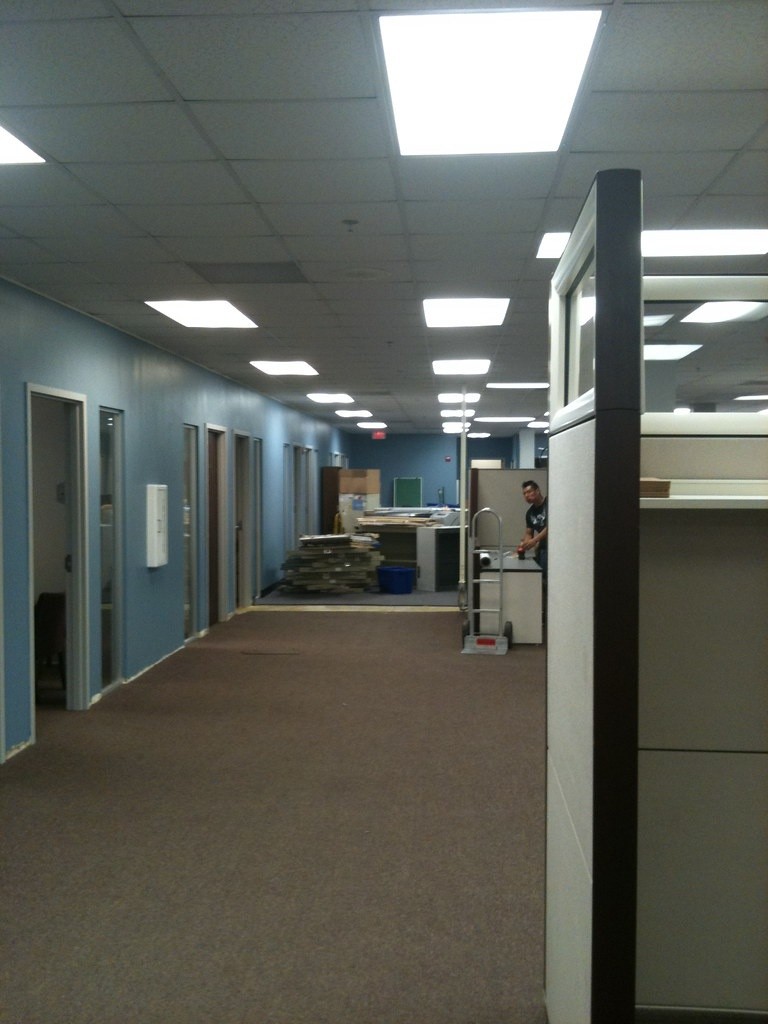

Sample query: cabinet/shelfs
[416,527,467,593]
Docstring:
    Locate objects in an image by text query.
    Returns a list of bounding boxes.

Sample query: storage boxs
[378,566,415,596]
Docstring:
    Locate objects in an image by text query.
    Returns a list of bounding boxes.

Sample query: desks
[479,546,543,644]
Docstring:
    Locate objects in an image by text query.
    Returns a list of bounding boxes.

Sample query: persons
[515,480,549,627]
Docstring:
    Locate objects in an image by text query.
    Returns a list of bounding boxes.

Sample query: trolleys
[461,506,513,655]
